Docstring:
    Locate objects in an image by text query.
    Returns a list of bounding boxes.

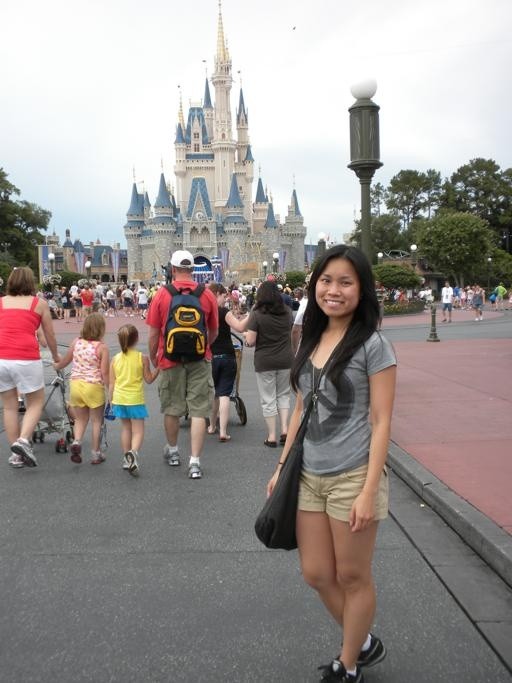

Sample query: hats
[171,248,195,268]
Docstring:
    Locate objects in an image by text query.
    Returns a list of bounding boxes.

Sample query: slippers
[280,434,287,442]
[264,438,278,447]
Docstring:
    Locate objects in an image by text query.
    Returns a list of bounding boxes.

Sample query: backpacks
[164,280,208,364]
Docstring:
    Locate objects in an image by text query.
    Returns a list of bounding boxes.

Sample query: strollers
[32,342,75,454]
[185,332,248,426]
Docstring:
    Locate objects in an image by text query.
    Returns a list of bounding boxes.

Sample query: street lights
[317,232,326,251]
[347,72,382,261]
[263,261,267,276]
[487,255,492,295]
[410,244,417,298]
[377,252,383,264]
[272,252,280,275]
[84,260,92,278]
[47,252,56,274]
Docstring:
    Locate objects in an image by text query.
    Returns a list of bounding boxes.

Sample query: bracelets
[279,461,285,465]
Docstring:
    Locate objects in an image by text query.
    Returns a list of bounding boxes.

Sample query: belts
[212,353,235,359]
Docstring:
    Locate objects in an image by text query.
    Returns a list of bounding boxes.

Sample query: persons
[0,266,62,469]
[53,312,109,464]
[144,250,218,479]
[0,248,510,446]
[109,324,160,473]
[267,245,387,683]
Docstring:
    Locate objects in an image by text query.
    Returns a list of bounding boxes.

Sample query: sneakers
[187,462,204,478]
[316,654,363,683]
[355,633,388,669]
[164,442,180,466]
[10,437,39,467]
[122,449,141,477]
[70,439,83,462]
[8,452,25,467]
[89,448,106,464]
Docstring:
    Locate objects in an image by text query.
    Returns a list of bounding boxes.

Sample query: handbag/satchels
[254,439,304,549]
